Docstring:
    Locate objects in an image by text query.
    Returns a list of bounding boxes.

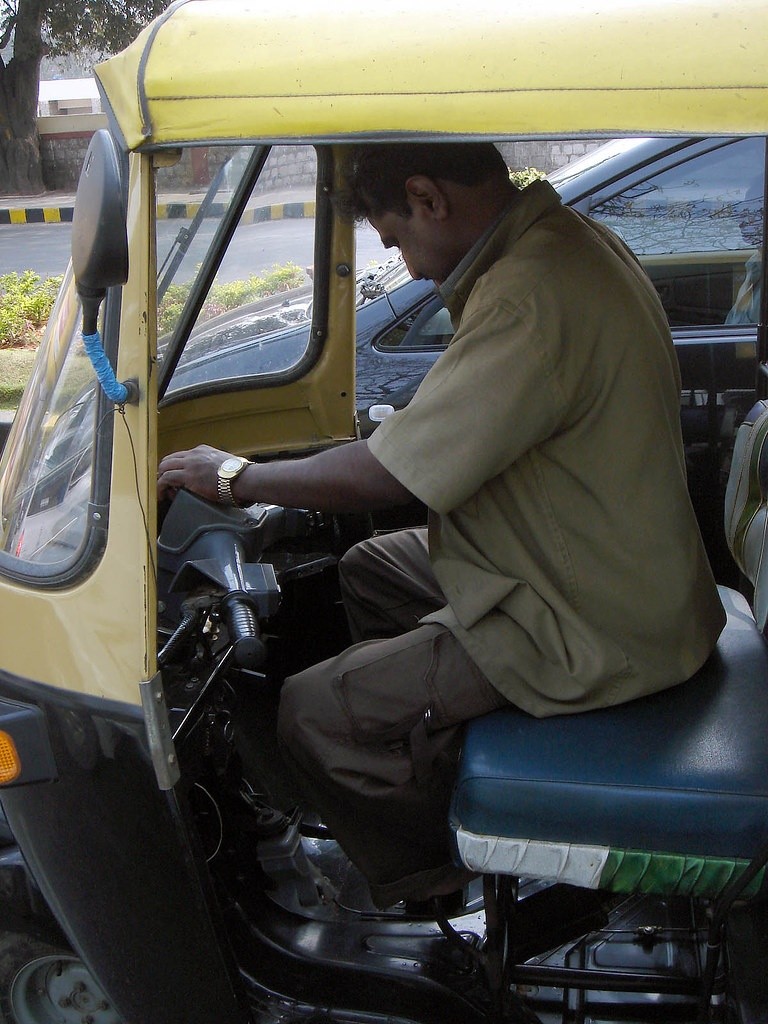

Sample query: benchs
[445,401,768,901]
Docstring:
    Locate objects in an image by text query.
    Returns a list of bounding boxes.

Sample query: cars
[12,138,768,534]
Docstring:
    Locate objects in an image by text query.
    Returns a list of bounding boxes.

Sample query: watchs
[216,457,261,511]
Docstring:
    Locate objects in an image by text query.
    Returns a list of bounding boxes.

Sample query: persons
[154,147,726,929]
